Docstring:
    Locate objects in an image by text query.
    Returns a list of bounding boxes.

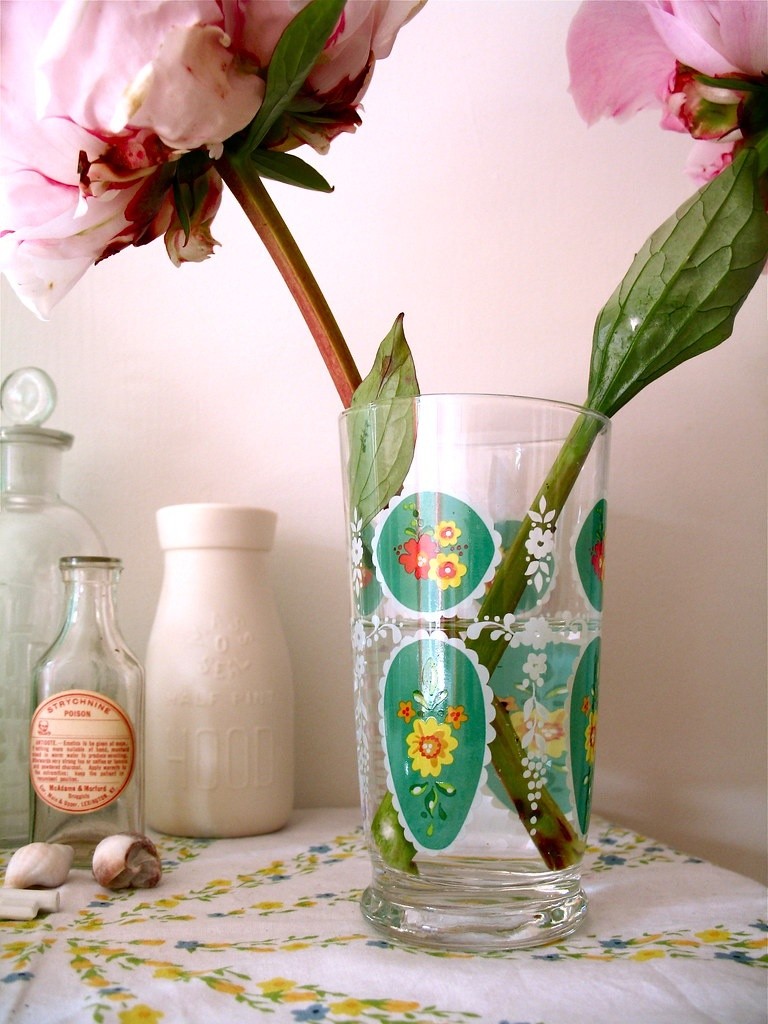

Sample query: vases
[334,396,611,953]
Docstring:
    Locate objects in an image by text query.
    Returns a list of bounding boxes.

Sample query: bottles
[0,365,115,847]
[29,554,147,866]
[143,503,297,837]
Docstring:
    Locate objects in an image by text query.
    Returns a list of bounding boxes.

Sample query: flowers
[0,0,767,328]
